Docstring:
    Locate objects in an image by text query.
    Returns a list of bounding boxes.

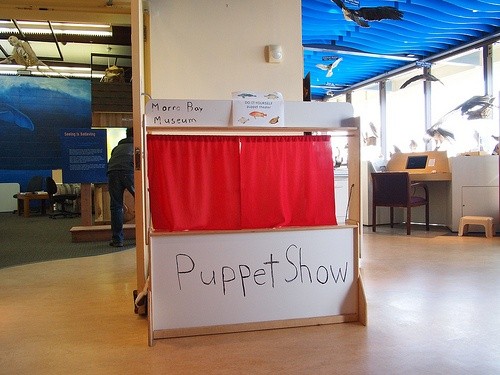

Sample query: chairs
[371,172,430,236]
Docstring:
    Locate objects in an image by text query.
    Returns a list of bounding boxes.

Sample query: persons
[106,127,135,247]
[0,36,46,65]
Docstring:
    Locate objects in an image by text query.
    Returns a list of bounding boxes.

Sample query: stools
[457,215,496,237]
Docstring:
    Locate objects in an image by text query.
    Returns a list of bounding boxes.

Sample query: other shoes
[110,239,124,247]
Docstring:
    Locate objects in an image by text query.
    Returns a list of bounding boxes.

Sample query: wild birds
[332,0,406,26]
[361,94,496,153]
[315,57,342,79]
[400,73,444,90]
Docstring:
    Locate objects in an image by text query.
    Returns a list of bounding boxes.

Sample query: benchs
[13,192,49,218]
